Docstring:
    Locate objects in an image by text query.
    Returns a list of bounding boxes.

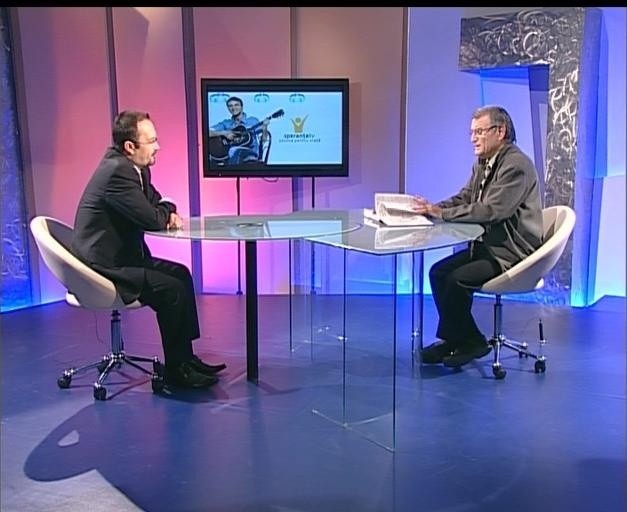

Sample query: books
[364,192,432,228]
[365,218,434,251]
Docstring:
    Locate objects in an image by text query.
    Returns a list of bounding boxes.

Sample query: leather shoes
[166,367,220,390]
[191,355,226,376]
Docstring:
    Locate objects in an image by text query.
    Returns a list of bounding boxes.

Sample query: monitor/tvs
[201,77,350,178]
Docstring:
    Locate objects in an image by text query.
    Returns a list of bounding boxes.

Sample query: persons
[411,103,545,368]
[210,96,269,164]
[72,110,226,391]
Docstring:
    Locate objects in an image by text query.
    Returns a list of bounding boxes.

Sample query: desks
[287,206,484,455]
[142,210,361,383]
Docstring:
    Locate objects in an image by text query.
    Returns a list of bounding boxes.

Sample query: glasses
[132,137,158,145]
[469,125,500,135]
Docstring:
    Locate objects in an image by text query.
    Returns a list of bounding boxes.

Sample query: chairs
[447,204,575,379]
[29,215,166,401]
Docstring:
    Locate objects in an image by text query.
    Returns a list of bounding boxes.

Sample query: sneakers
[443,334,489,367]
[423,339,454,363]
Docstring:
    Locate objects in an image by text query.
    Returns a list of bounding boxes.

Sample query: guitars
[208,110,284,162]
